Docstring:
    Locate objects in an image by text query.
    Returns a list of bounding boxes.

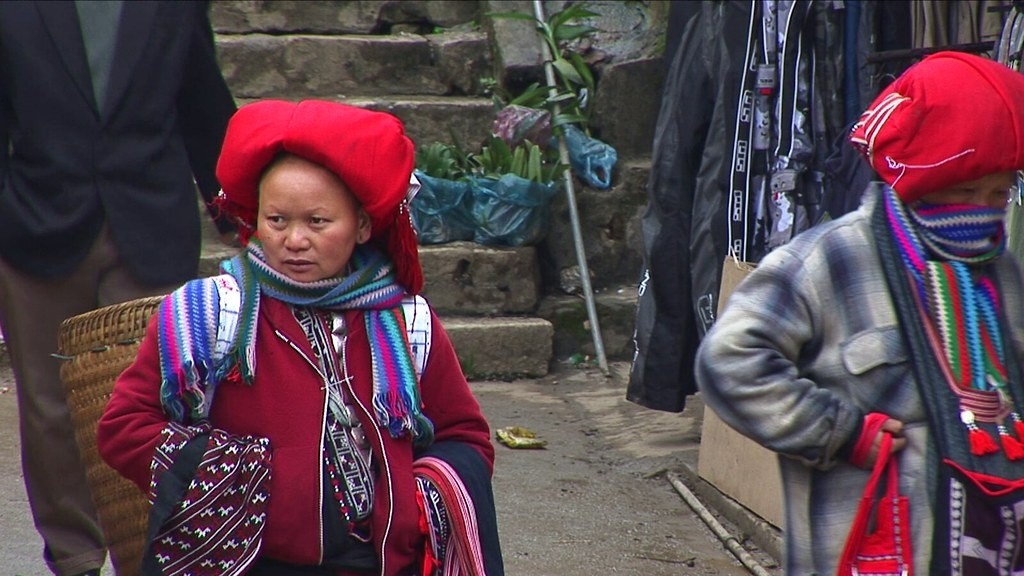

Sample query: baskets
[56,294,170,576]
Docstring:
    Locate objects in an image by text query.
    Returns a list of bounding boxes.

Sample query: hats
[848,50,1024,201]
[215,100,423,295]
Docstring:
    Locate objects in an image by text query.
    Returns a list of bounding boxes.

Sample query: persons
[98,98,496,576]
[696,53,1024,576]
[0,0,240,576]
